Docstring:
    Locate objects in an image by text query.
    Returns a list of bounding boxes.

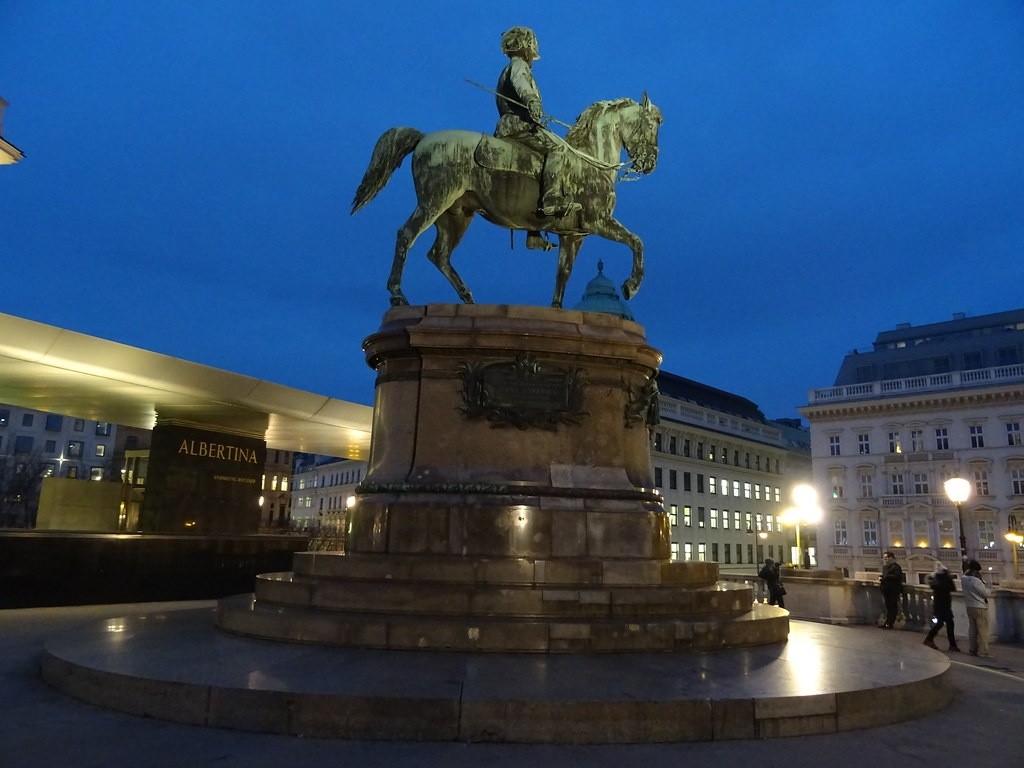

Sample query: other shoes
[949,646,961,652]
[923,639,939,650]
[978,653,994,658]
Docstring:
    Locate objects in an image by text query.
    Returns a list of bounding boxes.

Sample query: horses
[350,90,663,309]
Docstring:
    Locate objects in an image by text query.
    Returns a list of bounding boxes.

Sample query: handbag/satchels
[775,580,787,596]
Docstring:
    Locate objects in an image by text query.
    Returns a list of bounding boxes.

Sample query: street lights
[746,516,767,574]
[784,485,817,569]
[944,478,970,588]
[1005,530,1023,579]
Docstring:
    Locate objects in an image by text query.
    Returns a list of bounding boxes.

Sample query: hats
[969,560,981,571]
[935,561,948,575]
[764,558,774,565]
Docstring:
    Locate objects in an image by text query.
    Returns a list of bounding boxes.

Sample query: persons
[759,559,785,610]
[923,560,961,652]
[961,558,996,659]
[491,24,582,250]
[878,551,903,630]
